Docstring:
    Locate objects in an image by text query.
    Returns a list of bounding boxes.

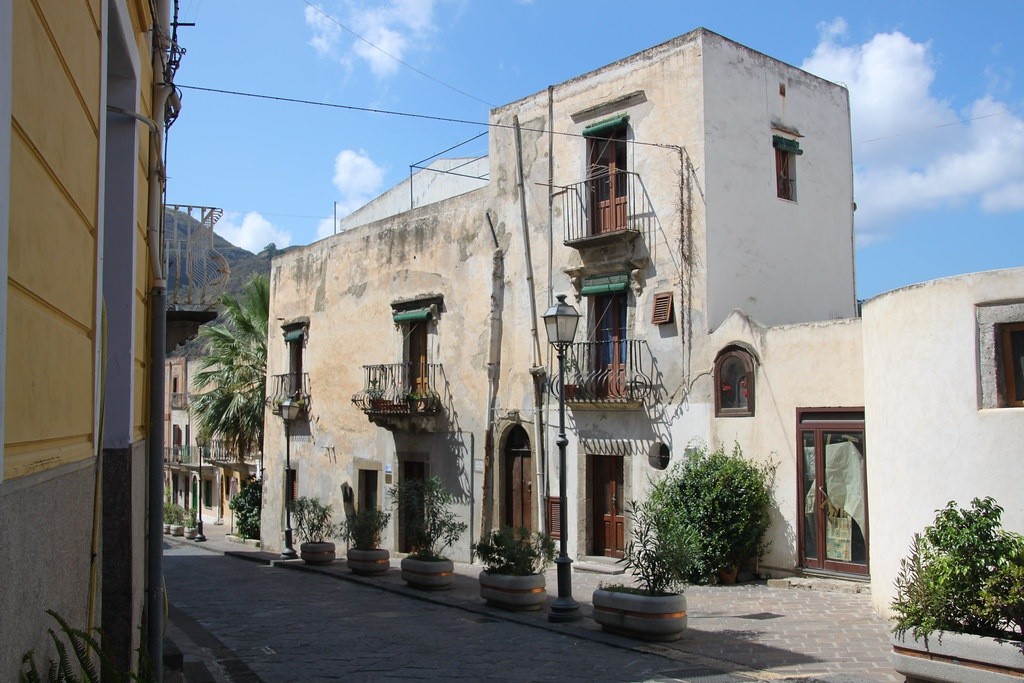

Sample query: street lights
[543,295,586,623]
[280,397,301,559]
[195,432,207,543]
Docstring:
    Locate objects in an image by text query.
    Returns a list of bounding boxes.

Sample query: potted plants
[184,508,198,539]
[163,503,171,534]
[276,399,284,412]
[386,476,467,591]
[338,509,392,577]
[888,496,1024,683]
[469,526,557,611]
[297,400,305,410]
[593,473,703,642]
[290,497,336,565]
[168,504,184,535]
[647,436,780,580]
